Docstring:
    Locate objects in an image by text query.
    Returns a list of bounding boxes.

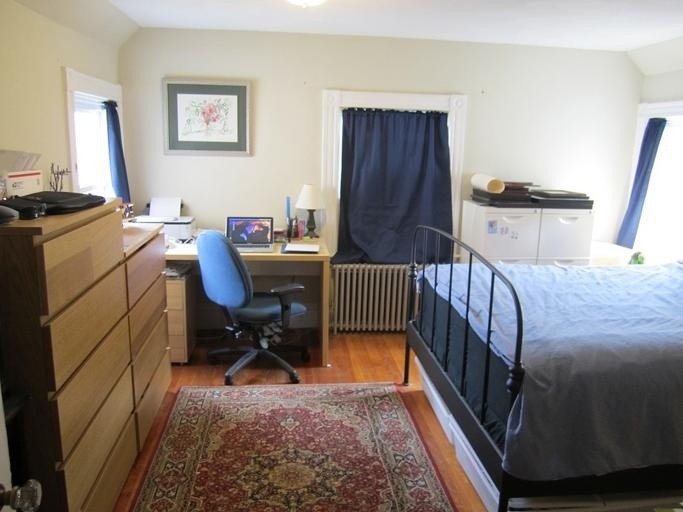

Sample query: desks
[162,236,332,368]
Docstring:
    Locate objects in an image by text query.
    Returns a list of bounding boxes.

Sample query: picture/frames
[160,78,251,157]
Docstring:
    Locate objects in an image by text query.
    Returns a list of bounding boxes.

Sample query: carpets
[123,382,457,512]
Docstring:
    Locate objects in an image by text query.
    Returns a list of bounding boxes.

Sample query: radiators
[330,262,449,333]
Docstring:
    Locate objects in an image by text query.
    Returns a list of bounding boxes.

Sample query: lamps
[295,185,325,238]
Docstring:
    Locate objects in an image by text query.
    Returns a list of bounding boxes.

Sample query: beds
[401,225,683,512]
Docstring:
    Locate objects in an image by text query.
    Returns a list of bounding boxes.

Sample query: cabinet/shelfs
[463,198,594,266]
[165,265,198,365]
[0,195,174,510]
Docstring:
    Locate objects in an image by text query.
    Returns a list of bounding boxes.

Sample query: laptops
[225,217,274,253]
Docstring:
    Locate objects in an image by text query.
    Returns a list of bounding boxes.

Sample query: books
[280,243,318,253]
[166,262,191,279]
[470,176,593,209]
[284,243,320,252]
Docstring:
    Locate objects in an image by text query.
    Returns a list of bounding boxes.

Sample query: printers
[133,197,197,240]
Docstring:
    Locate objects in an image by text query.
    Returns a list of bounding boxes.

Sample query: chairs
[193,230,310,388]
